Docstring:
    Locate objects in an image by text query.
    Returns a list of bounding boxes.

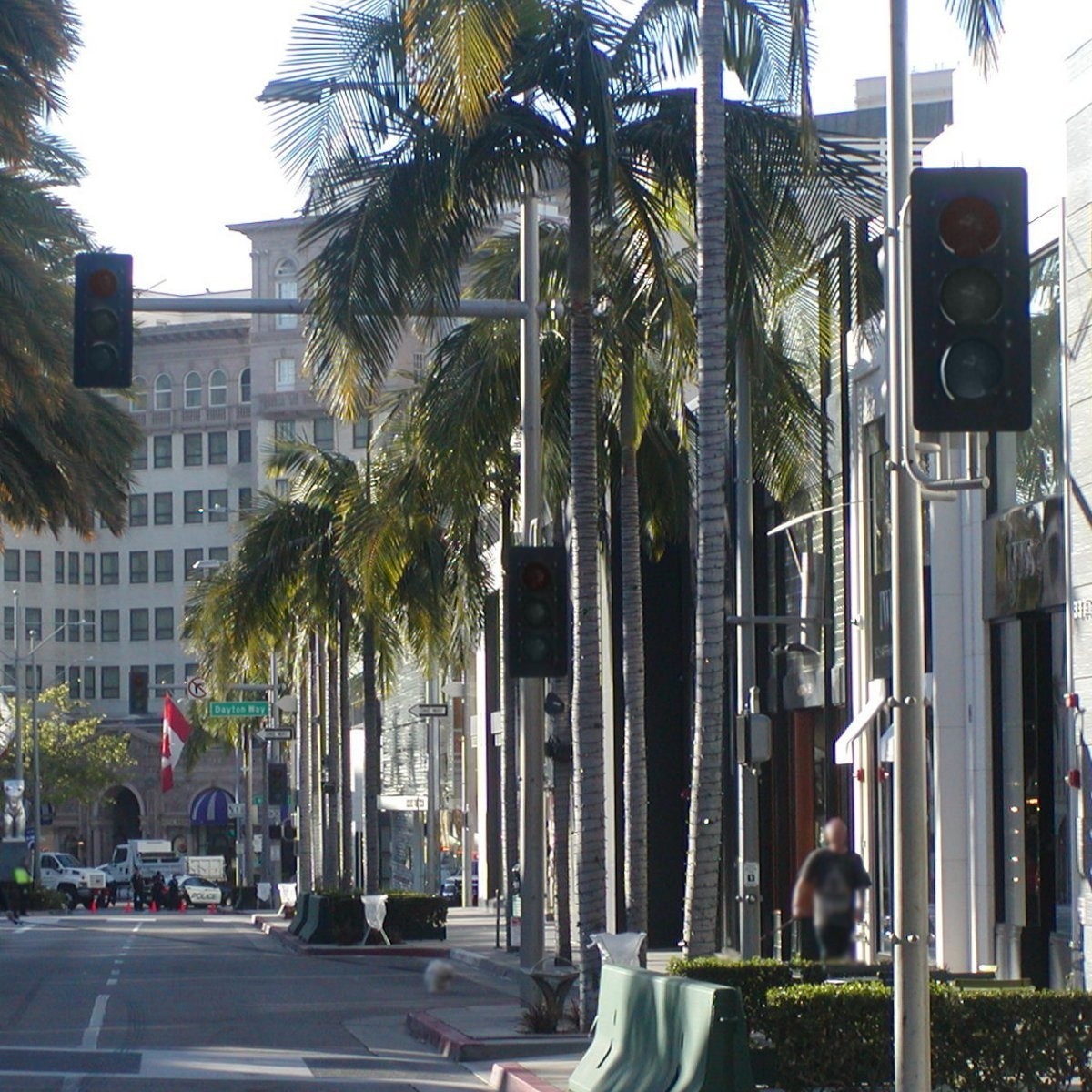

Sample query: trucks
[91,839,228,903]
[30,848,108,912]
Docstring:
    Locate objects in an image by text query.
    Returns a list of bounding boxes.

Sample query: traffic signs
[410,703,448,717]
[255,728,294,741]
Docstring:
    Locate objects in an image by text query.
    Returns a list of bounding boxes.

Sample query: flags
[162,689,190,791]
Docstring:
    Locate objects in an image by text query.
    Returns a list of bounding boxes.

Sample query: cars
[143,874,234,911]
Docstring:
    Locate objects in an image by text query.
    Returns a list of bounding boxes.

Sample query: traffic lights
[268,824,281,840]
[268,763,289,805]
[506,543,570,679]
[912,163,1035,436]
[74,252,134,389]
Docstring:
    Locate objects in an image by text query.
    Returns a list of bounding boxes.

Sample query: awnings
[189,787,234,823]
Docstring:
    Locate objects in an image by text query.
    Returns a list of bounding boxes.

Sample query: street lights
[197,505,355,890]
[259,76,552,1012]
[194,559,286,884]
[3,588,96,844]
[28,628,95,892]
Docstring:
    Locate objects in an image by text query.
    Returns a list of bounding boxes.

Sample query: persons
[131,867,145,911]
[792,818,873,961]
[151,870,165,911]
[14,856,32,916]
[168,875,179,910]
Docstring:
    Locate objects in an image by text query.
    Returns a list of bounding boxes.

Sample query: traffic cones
[89,898,98,913]
[180,899,187,913]
[150,901,157,912]
[209,900,216,913]
[125,902,132,913]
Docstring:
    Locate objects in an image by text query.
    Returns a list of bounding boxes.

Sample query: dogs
[423,958,452,993]
